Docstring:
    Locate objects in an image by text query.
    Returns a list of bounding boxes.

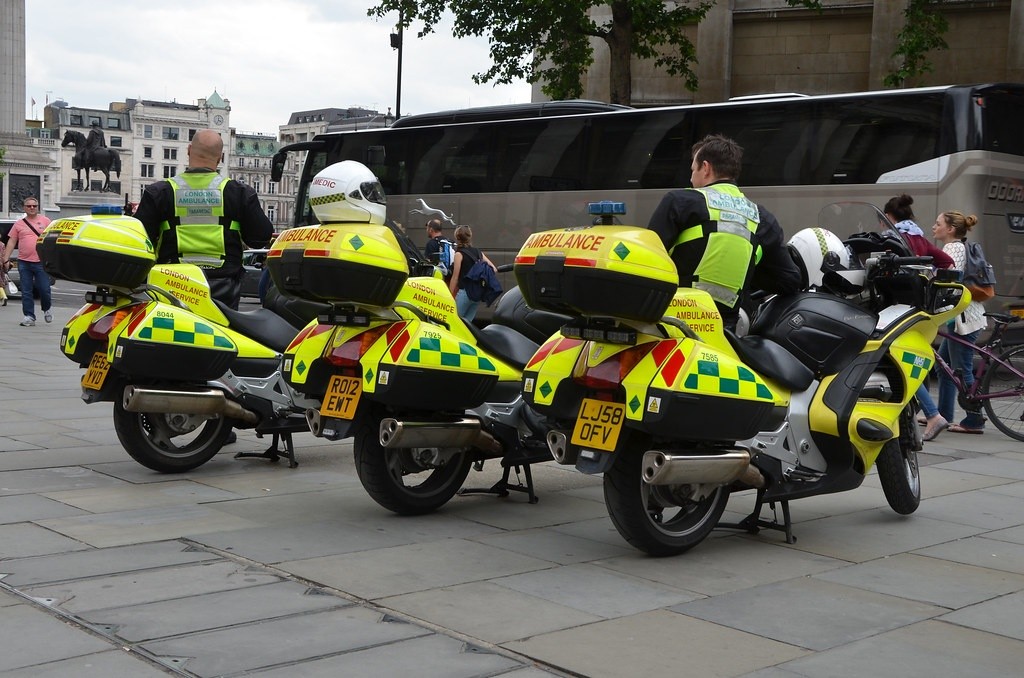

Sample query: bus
[266,83,1019,340]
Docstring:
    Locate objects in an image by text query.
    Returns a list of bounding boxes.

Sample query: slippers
[946,422,984,434]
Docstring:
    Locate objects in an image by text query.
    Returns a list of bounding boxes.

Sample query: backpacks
[434,236,458,278]
[953,239,996,301]
[458,246,503,306]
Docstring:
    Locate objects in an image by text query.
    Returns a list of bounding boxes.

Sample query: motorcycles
[35,214,445,474]
[512,196,972,555]
[266,222,575,516]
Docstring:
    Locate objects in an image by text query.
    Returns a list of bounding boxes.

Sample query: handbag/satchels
[4,273,18,296]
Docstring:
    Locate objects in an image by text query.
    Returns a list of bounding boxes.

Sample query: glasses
[24,205,38,208]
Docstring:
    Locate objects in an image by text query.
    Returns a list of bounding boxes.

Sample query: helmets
[308,160,388,225]
[785,227,850,295]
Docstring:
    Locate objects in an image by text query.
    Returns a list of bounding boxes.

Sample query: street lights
[376,1,404,125]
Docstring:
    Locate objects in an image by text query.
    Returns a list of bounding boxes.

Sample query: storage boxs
[266,223,410,308]
[35,214,157,290]
[512,225,680,325]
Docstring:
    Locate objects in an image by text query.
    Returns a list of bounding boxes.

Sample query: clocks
[214,115,224,126]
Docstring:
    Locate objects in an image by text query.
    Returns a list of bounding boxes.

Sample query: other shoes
[2,297,8,306]
[921,417,949,440]
[44,310,52,323]
[918,416,927,424]
[19,315,35,326]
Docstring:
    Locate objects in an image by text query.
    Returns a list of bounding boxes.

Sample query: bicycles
[911,312,1020,438]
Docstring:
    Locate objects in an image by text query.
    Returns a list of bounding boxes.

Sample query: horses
[61,129,121,192]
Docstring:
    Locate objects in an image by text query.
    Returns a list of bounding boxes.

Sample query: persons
[74,120,105,170]
[647,134,804,332]
[132,128,274,445]
[0,197,53,326]
[424,219,498,323]
[878,194,990,439]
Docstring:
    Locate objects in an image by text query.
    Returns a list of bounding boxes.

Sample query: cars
[240,248,270,297]
